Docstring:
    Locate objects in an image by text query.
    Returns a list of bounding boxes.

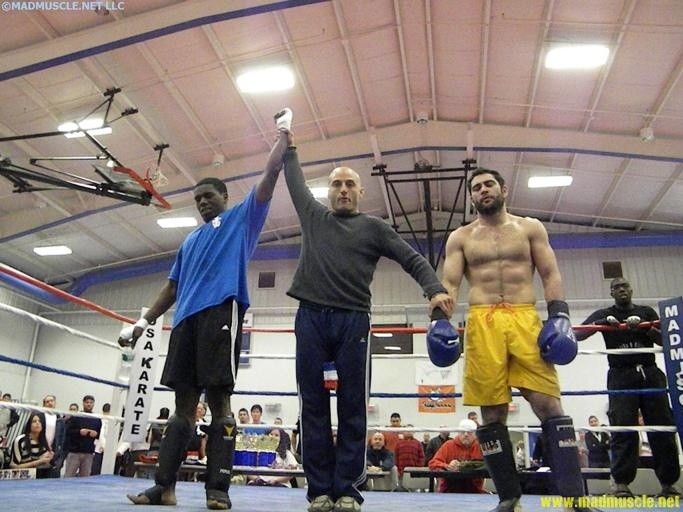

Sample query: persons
[11,410,60,478]
[146,408,170,442]
[468,412,478,424]
[366,432,396,472]
[291,418,301,464]
[44,396,63,462]
[90,404,109,475]
[426,426,452,465]
[245,405,271,436]
[238,409,249,424]
[246,428,297,488]
[276,129,454,512]
[186,401,208,460]
[394,425,424,481]
[584,416,611,480]
[430,419,488,493]
[272,419,283,430]
[0,390,19,435]
[575,277,680,497]
[63,396,102,478]
[69,404,79,412]
[383,413,407,452]
[426,168,578,510]
[116,108,295,507]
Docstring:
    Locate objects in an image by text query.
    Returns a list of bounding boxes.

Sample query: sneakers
[334,496,361,511]
[308,496,332,510]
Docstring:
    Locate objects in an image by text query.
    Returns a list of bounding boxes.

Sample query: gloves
[427,308,462,367]
[119,319,148,345]
[537,302,578,366]
[276,108,293,131]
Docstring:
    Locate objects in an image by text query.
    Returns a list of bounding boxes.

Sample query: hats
[456,419,478,433]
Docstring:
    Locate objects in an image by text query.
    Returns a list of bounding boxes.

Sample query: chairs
[580,468,616,497]
[628,469,663,496]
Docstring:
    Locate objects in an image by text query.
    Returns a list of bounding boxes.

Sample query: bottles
[119,343,133,384]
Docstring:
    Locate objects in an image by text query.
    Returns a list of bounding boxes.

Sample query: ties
[596,431,599,440]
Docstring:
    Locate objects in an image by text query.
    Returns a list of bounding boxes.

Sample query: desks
[133,463,389,488]
[404,467,610,492]
